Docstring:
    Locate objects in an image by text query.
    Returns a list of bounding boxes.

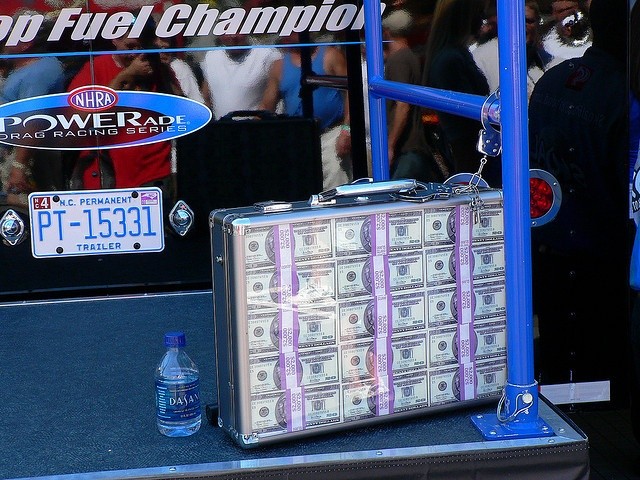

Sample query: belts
[320,120,343,135]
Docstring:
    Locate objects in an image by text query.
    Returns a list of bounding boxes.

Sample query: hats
[381,9,413,32]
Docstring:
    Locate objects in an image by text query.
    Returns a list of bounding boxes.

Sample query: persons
[378,9,427,182]
[538,0,593,72]
[255,20,355,192]
[153,30,206,173]
[67,7,184,207]
[0,43,72,196]
[201,19,284,121]
[355,32,385,179]
[525,0,544,102]
[441,1,491,187]
[527,0,639,407]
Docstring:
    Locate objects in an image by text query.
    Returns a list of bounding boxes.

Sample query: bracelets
[337,123,352,131]
[340,130,351,137]
[11,161,26,171]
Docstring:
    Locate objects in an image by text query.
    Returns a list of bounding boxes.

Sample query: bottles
[154,330,202,439]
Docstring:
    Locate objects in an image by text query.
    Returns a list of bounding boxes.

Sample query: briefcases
[208,177,508,450]
[177,110,323,248]
